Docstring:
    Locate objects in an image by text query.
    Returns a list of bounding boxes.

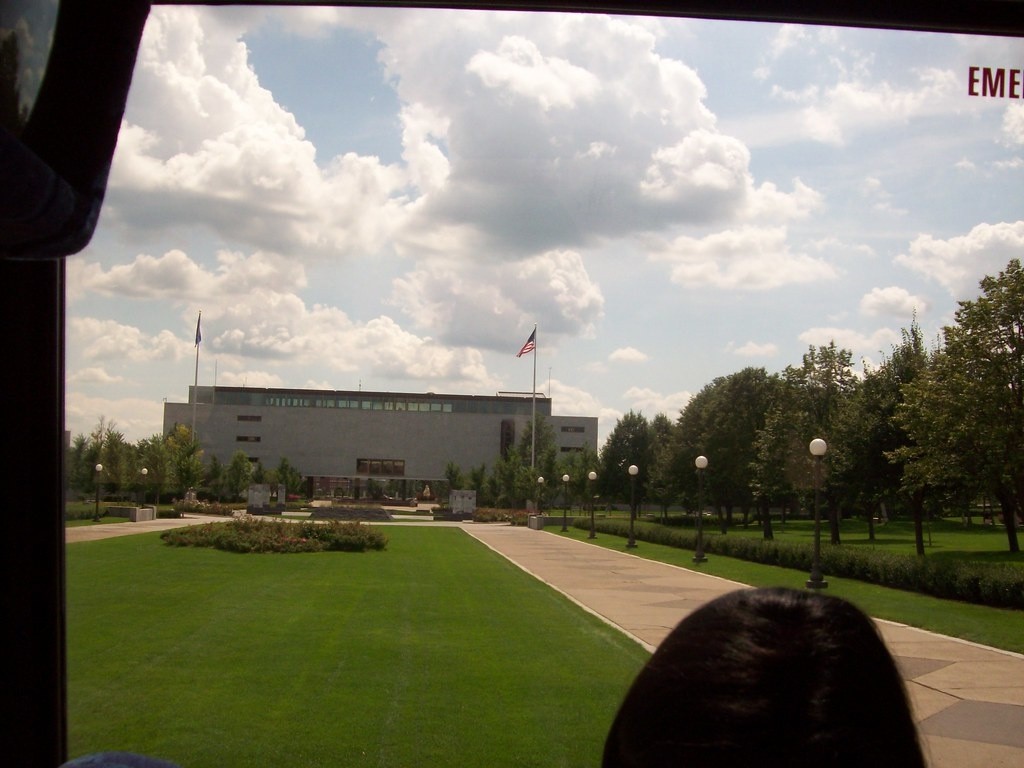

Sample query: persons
[600,585,934,768]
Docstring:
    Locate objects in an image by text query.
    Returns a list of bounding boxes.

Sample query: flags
[195,315,201,346]
[516,329,536,357]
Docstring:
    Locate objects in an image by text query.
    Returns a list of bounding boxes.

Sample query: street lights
[807,437,828,589]
[538,476,544,515]
[92,463,104,522]
[626,465,639,549]
[560,475,570,531]
[586,471,599,540]
[141,468,148,508]
[690,455,710,563]
